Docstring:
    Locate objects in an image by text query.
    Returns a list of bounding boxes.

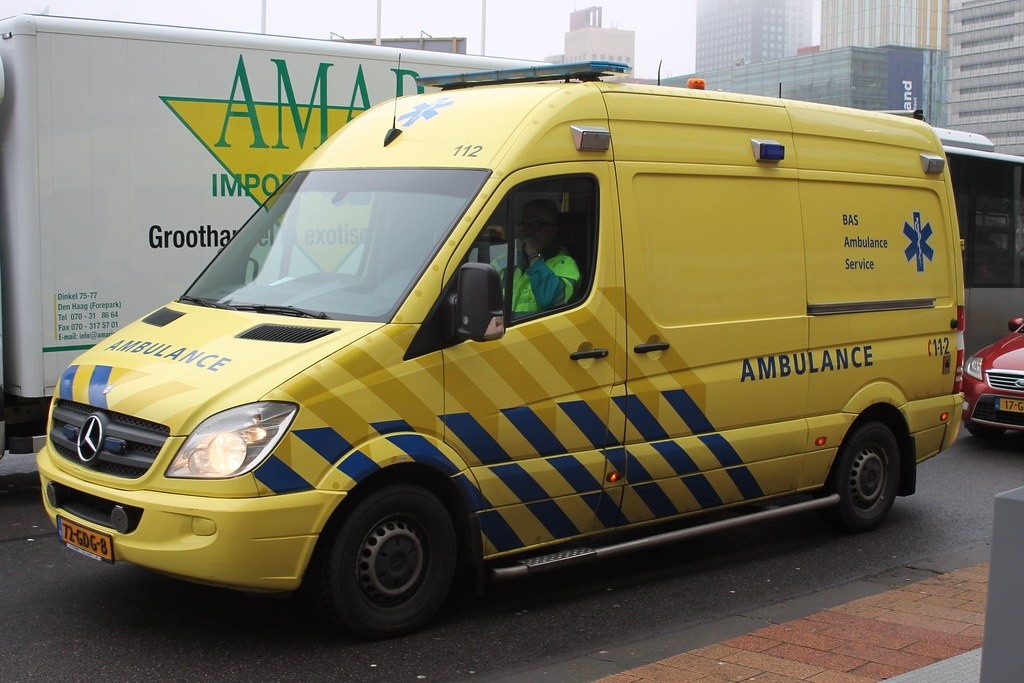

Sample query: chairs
[557,211,595,282]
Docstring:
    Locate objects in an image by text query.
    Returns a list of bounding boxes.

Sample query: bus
[928,125,1024,363]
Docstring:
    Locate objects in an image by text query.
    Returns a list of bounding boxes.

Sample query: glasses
[518,220,556,230]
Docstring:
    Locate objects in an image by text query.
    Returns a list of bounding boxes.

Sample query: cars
[962,311,1024,441]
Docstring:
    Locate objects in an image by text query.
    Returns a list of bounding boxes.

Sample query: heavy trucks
[0,12,1024,481]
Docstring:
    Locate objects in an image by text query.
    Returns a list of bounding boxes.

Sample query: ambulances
[33,59,968,636]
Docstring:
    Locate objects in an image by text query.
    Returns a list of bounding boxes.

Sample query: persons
[490,199,578,313]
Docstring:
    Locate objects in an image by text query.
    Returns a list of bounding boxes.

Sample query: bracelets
[528,253,544,260]
